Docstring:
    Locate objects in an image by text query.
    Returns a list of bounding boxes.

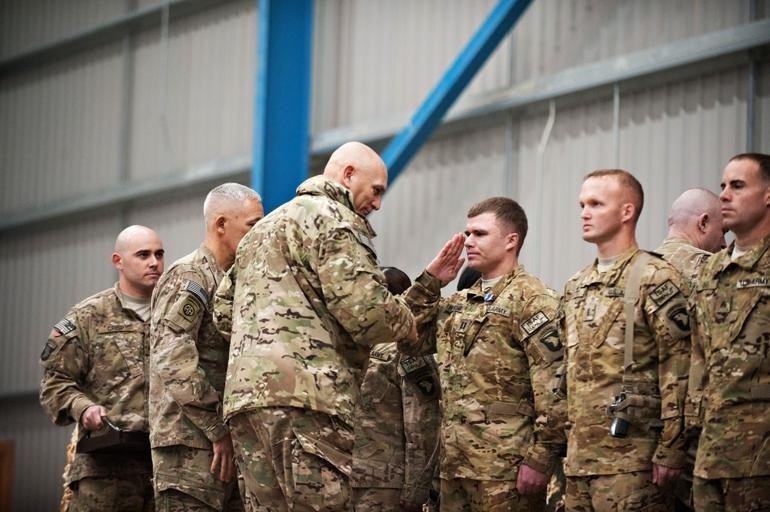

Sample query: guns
[610,392,661,438]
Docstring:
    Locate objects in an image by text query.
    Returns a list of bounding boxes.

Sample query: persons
[455,266,478,288]
[650,185,731,511]
[212,141,418,511]
[148,182,265,510]
[350,267,434,511]
[397,196,565,512]
[683,153,769,512]
[557,169,697,512]
[37,226,164,511]
